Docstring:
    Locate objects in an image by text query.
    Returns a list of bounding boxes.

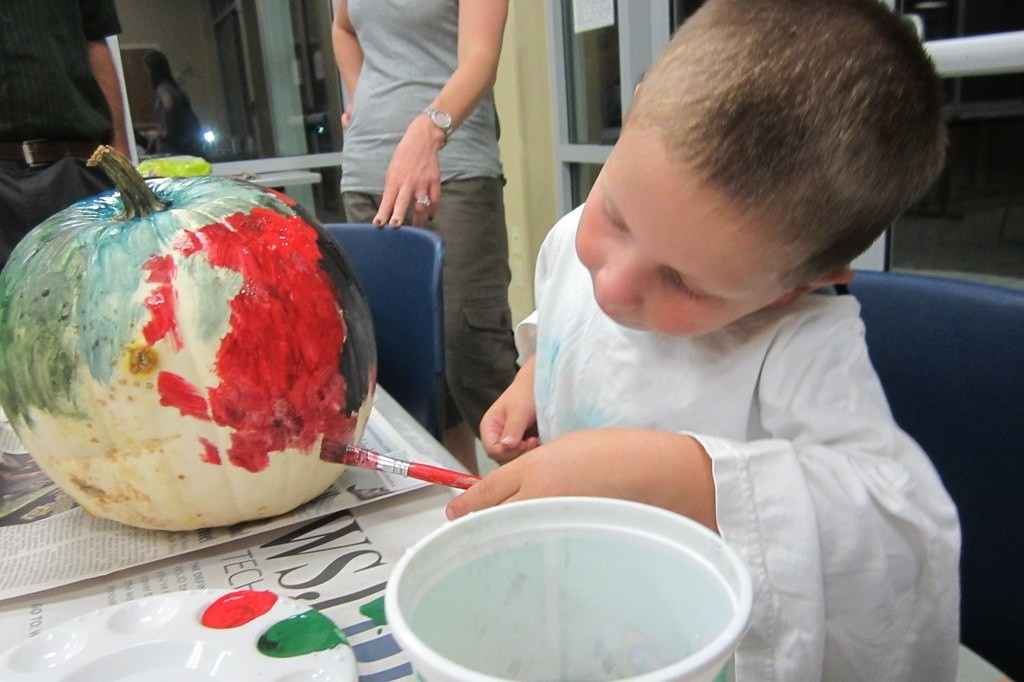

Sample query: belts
[0,136,107,168]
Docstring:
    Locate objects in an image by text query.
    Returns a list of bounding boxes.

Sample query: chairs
[323,218,446,446]
[845,265,1024,682]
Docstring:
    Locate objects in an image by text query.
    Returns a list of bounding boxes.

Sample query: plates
[0,589,358,682]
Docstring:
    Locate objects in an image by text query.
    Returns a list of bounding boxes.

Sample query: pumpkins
[0,143,379,530]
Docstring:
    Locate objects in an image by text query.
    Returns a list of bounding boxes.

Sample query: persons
[143,52,184,154]
[0,0,132,273]
[445,0,962,682]
[331,0,539,476]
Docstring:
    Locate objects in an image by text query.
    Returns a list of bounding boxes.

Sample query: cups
[384,495,753,682]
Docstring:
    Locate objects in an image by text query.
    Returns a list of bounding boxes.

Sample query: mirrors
[108,0,348,180]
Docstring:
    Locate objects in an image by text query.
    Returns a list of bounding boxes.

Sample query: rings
[417,197,431,206]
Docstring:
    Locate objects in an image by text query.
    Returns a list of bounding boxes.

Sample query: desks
[238,168,323,195]
[0,378,487,682]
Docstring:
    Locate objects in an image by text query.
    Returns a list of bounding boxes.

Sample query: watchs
[423,107,454,136]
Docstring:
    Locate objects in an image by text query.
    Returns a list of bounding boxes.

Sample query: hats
[142,52,180,90]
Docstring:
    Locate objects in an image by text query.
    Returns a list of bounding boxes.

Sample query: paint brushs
[320,437,480,489]
